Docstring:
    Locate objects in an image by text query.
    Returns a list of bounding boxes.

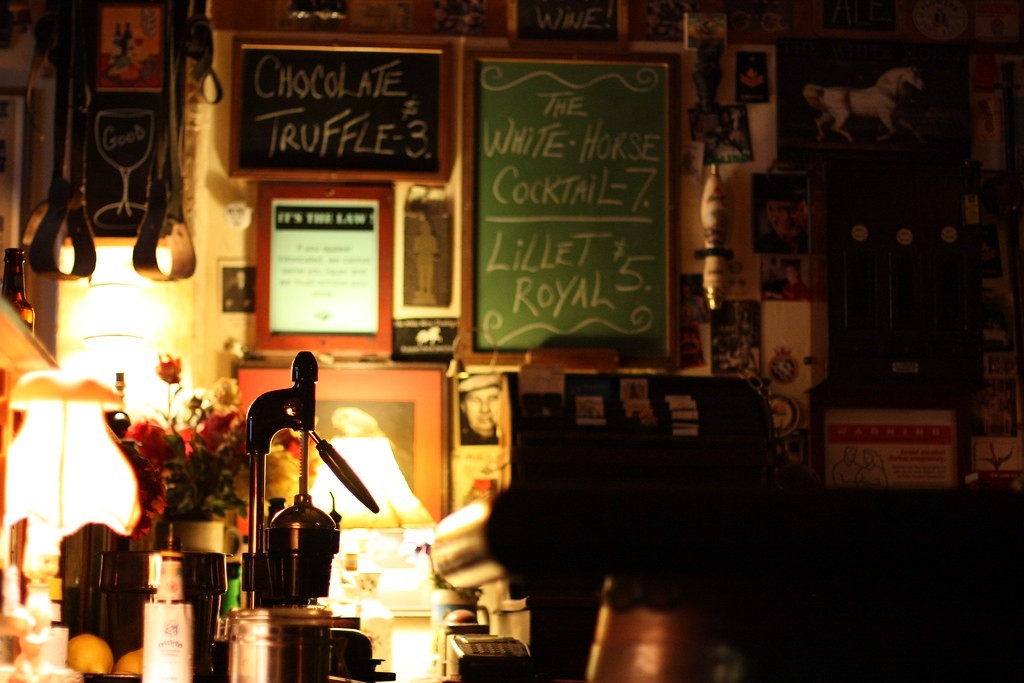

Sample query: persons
[458,376,500,444]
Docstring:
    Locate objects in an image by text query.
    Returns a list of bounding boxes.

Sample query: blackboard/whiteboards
[226,29,449,186]
[457,39,683,374]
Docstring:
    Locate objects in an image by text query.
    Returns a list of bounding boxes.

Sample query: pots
[216,608,332,683]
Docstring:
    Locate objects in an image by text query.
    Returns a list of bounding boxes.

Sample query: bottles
[144,536,194,683]
